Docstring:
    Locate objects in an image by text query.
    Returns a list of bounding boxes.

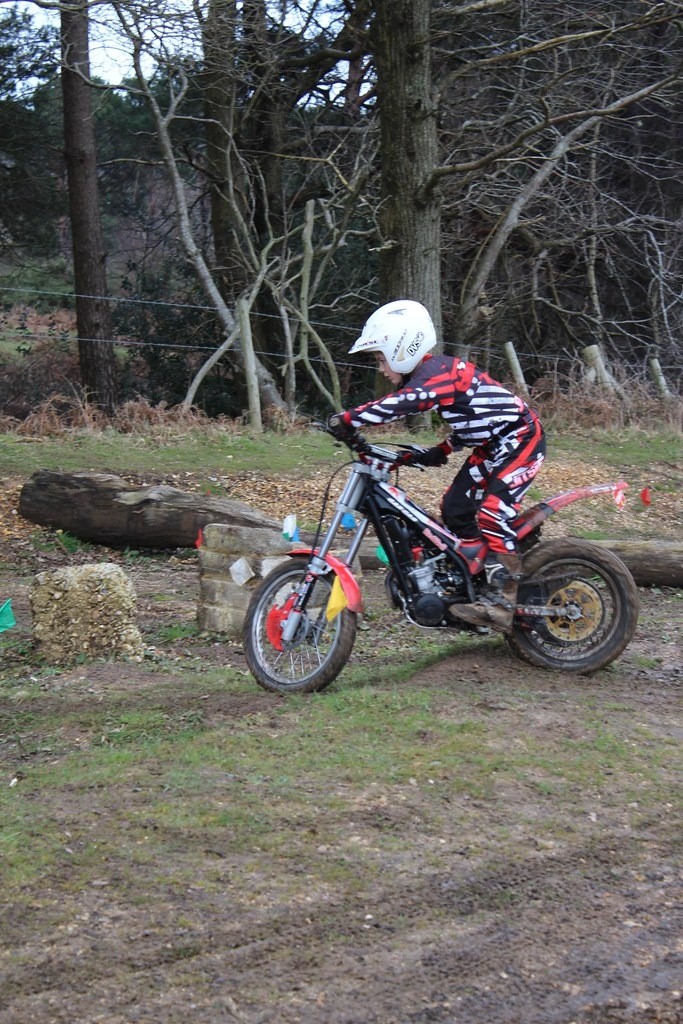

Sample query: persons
[327,300,548,636]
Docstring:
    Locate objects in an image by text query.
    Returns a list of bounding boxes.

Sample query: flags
[342,514,357,531]
[643,488,651,505]
[376,546,389,565]
[0,599,16,633]
[282,515,298,542]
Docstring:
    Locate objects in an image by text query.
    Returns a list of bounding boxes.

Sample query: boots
[448,553,523,635]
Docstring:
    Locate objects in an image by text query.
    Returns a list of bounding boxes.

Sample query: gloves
[413,446,446,466]
[331,412,353,439]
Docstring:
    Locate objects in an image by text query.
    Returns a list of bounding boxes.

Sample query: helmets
[348,300,437,374]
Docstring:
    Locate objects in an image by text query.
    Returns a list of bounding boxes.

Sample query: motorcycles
[240,410,641,696]
